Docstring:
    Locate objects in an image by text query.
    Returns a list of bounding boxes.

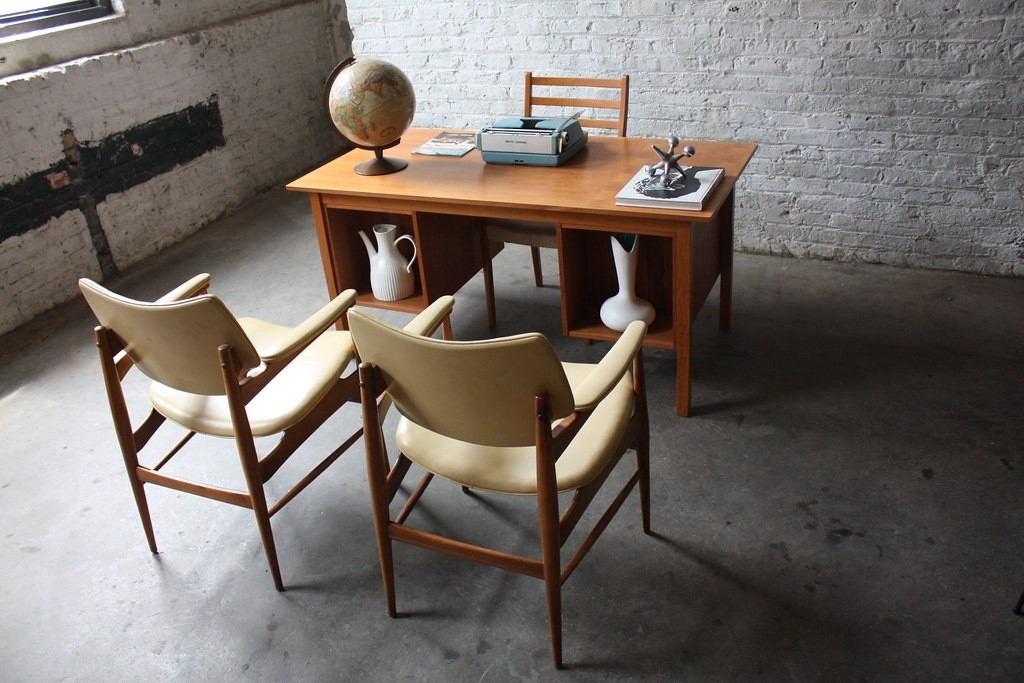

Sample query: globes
[322,56,417,176]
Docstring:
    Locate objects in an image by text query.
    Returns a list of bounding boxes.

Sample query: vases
[359,223,418,302]
[599,231,656,333]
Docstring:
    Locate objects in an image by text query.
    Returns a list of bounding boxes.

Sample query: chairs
[340,293,652,671]
[78,272,392,592]
[480,73,630,328]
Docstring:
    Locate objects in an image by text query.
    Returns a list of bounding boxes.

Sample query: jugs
[358,224,417,302]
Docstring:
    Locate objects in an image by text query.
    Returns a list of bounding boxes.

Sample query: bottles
[600,233,655,333]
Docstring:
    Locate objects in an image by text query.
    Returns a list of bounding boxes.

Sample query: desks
[285,129,761,416]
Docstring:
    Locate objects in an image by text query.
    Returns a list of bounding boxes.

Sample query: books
[411,131,476,157]
[615,164,725,211]
